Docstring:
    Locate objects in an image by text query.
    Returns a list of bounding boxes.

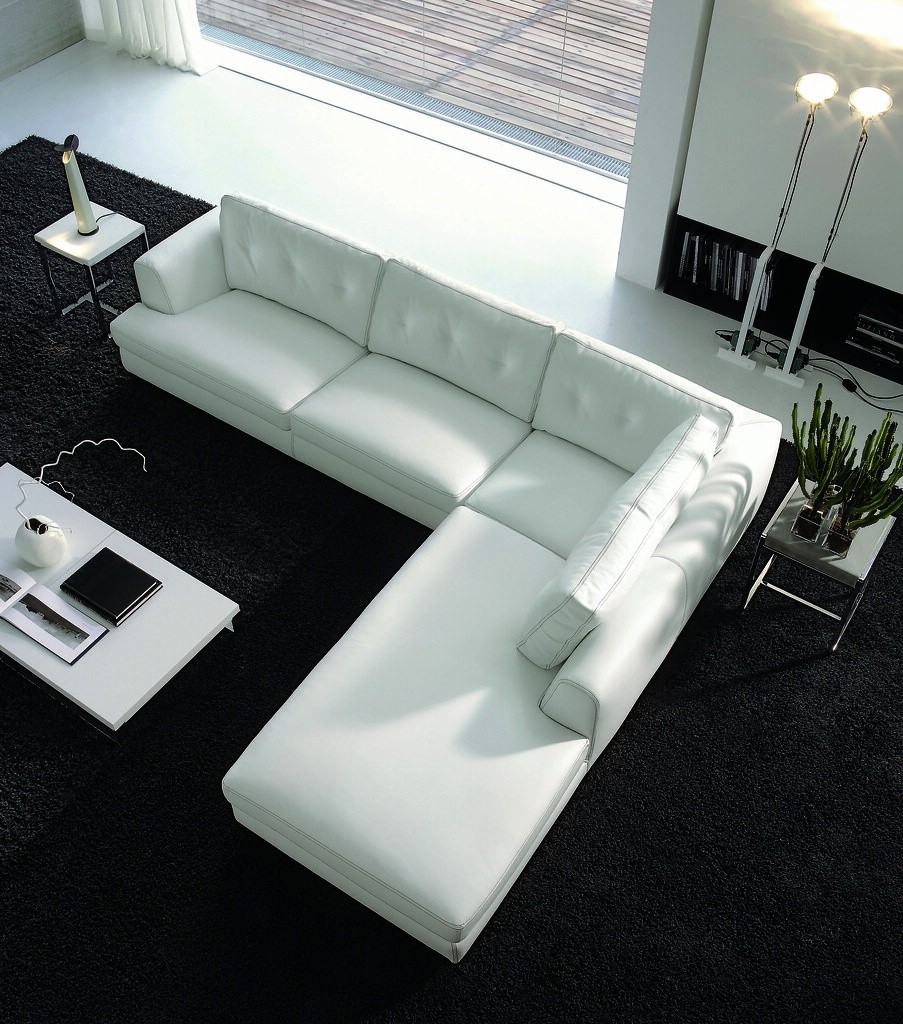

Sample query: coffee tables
[0,462,242,747]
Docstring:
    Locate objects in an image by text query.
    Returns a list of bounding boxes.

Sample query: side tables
[33,200,150,339]
[742,476,898,653]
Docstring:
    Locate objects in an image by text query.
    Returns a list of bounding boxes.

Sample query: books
[0,557,110,666]
[61,546,164,627]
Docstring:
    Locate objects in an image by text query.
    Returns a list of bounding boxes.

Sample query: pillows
[516,413,719,671]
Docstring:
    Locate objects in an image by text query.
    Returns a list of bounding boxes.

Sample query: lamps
[764,86,892,390]
[717,72,840,373]
[54,134,99,237]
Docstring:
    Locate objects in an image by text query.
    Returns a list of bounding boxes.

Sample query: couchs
[220,504,688,966]
[110,192,782,634]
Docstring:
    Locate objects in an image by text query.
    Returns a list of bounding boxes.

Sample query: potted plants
[789,382,865,547]
[822,414,903,562]
